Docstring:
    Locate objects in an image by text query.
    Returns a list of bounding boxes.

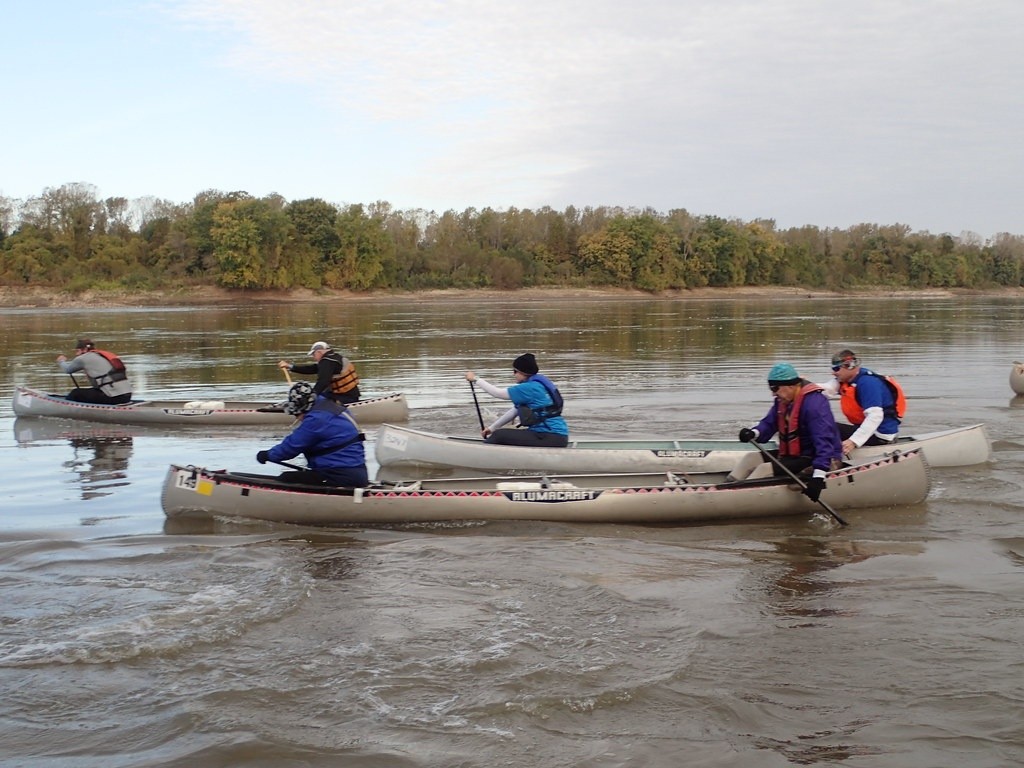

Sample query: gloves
[801,478,824,502]
[739,428,755,443]
[257,450,269,464]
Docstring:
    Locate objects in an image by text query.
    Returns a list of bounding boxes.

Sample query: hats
[512,354,539,376]
[307,341,329,356]
[832,350,854,365]
[75,340,95,349]
[288,382,316,415]
[767,363,799,385]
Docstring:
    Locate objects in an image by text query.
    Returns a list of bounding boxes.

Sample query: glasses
[514,369,518,373]
[770,385,779,392]
[832,366,841,372]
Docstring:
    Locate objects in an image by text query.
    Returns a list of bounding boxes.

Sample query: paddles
[282,365,294,388]
[469,379,488,439]
[69,372,79,389]
[746,431,850,526]
[268,459,311,472]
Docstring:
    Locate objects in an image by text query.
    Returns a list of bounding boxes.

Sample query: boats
[372,421,994,475]
[13,384,414,425]
[157,444,932,518]
[1009,359,1024,398]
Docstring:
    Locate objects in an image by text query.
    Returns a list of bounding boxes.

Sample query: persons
[463,353,567,447]
[722,363,842,503]
[278,341,361,404]
[816,349,906,454]
[56,340,132,405]
[257,382,368,487]
